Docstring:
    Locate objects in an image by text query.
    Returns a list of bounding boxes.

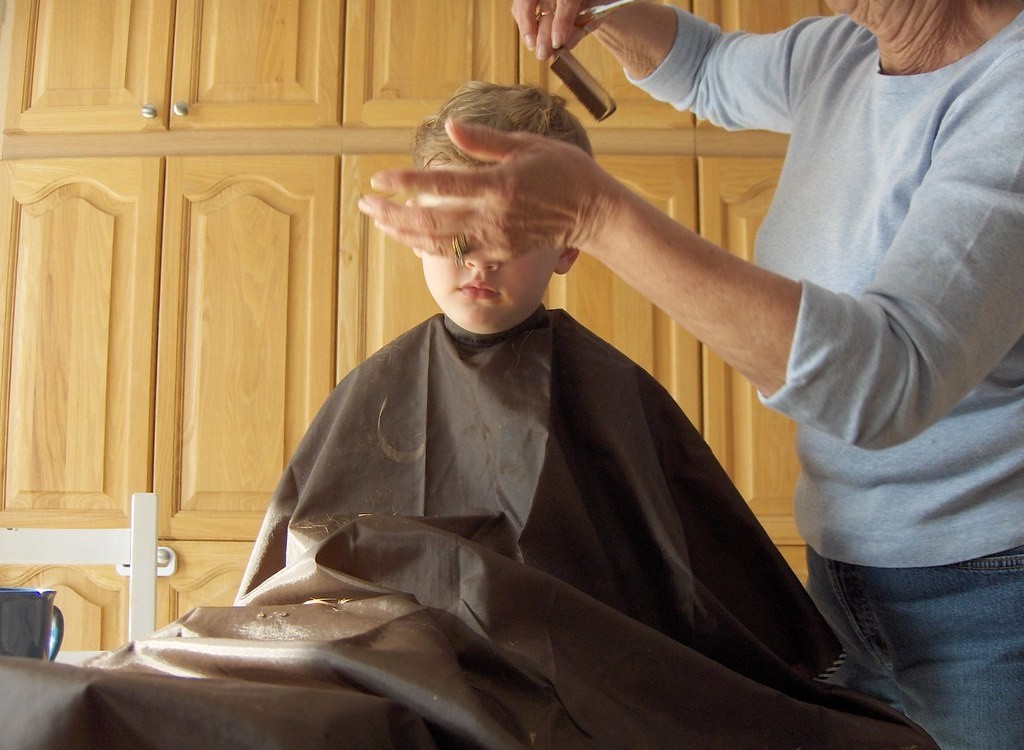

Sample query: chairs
[0,492,160,644]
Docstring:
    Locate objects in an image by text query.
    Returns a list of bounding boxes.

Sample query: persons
[413,79,599,338]
[356,0,1024,750]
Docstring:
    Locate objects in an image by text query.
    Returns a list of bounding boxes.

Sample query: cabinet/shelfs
[692,0,839,591]
[0,0,345,652]
[335,0,703,438]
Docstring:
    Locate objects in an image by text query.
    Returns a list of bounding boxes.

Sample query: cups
[0,587,64,662]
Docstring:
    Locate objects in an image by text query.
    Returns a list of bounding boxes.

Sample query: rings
[450,234,470,268]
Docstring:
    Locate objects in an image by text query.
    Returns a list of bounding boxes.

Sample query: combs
[550,46,617,122]
